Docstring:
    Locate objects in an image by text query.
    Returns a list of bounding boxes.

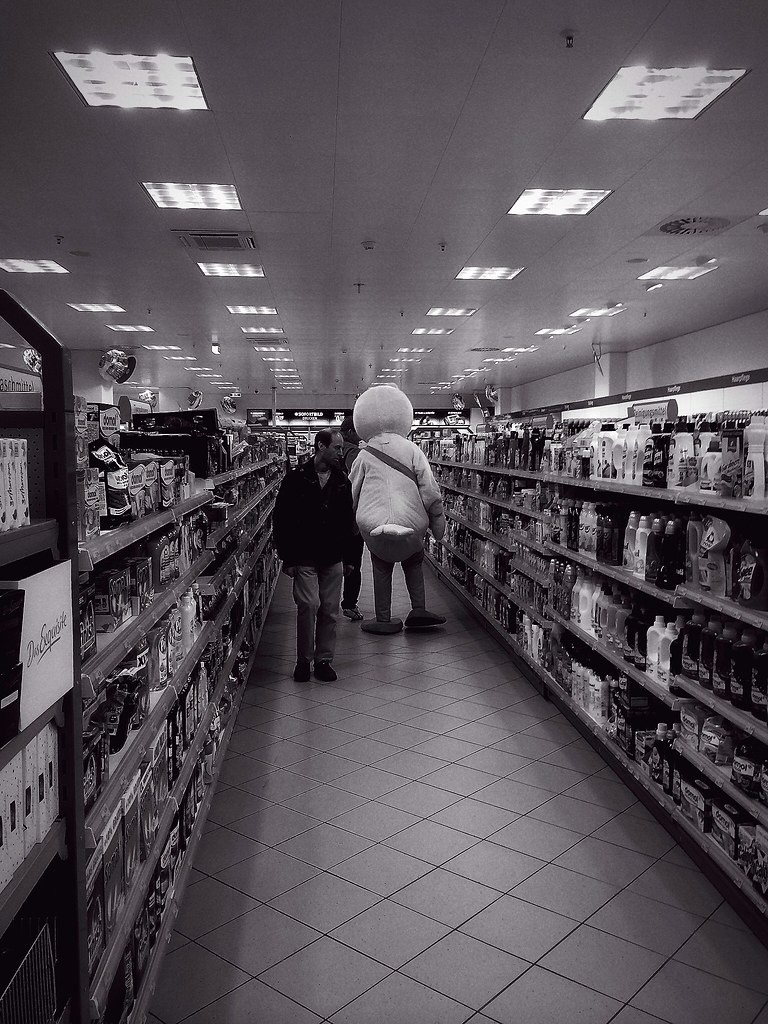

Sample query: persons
[341,416,364,620]
[273,429,356,682]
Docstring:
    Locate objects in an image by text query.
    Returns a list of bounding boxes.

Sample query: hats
[340,416,355,432]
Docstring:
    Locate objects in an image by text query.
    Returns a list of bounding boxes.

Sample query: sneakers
[294,659,310,682]
[313,660,338,682]
[343,605,363,620]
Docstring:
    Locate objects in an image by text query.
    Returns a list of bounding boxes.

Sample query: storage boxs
[0,438,74,900]
[634,703,768,904]
[74,395,222,1023]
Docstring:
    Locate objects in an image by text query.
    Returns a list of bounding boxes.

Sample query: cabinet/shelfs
[671,490,767,946]
[178,454,287,907]
[0,286,78,1024]
[425,456,671,834]
[72,489,179,1024]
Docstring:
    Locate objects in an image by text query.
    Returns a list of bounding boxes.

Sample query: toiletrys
[1,399,292,1021]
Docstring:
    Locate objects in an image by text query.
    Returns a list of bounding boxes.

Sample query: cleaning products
[409,418,765,911]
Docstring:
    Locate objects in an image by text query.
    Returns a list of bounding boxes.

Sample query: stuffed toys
[348,384,447,634]
